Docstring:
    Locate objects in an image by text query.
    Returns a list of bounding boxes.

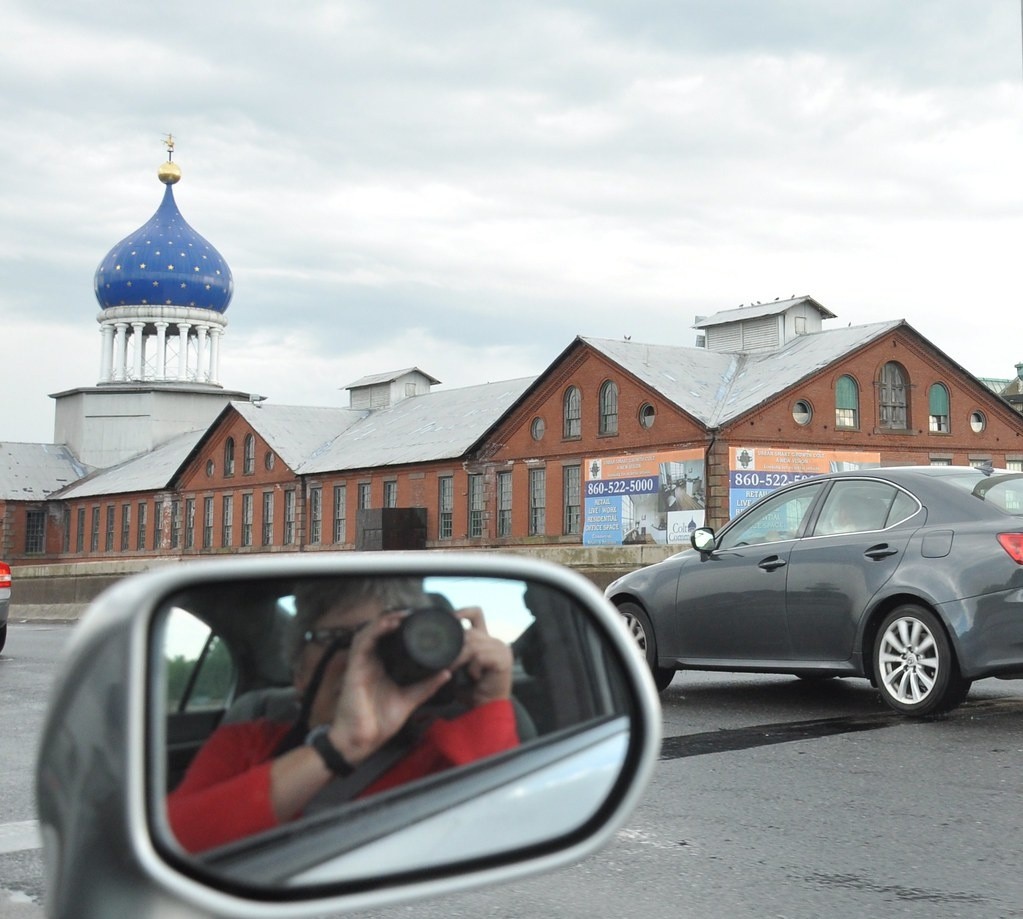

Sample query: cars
[161,575,630,890]
[604,459,1023,718]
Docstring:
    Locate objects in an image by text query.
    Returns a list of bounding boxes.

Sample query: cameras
[375,608,466,704]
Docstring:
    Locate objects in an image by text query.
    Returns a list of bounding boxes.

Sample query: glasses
[303,623,359,650]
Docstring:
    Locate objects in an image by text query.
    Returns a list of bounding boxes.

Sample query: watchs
[305,724,356,781]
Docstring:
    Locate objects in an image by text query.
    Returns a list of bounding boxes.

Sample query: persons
[166,572,520,855]
